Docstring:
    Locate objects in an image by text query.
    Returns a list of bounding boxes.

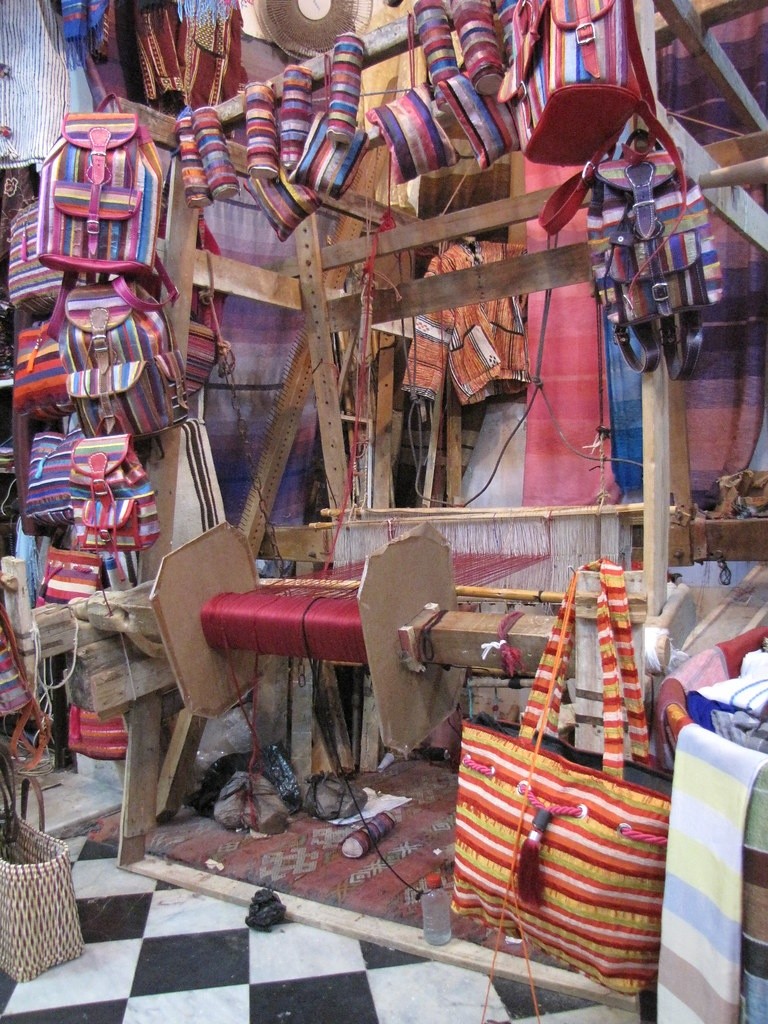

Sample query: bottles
[421,874,451,946]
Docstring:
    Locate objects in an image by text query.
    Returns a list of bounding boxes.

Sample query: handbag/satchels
[243,162,321,243]
[451,560,676,995]
[183,320,220,398]
[286,55,371,200]
[438,71,522,170]
[0,602,32,716]
[19,432,77,535]
[68,699,130,761]
[12,324,75,420]
[8,201,64,317]
[368,14,459,184]
[41,542,103,602]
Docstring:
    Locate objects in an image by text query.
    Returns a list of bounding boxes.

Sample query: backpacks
[35,94,163,278]
[59,279,190,439]
[69,416,160,583]
[585,133,712,381]
[497,0,661,167]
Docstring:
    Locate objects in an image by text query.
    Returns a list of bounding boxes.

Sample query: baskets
[1,744,84,984]
